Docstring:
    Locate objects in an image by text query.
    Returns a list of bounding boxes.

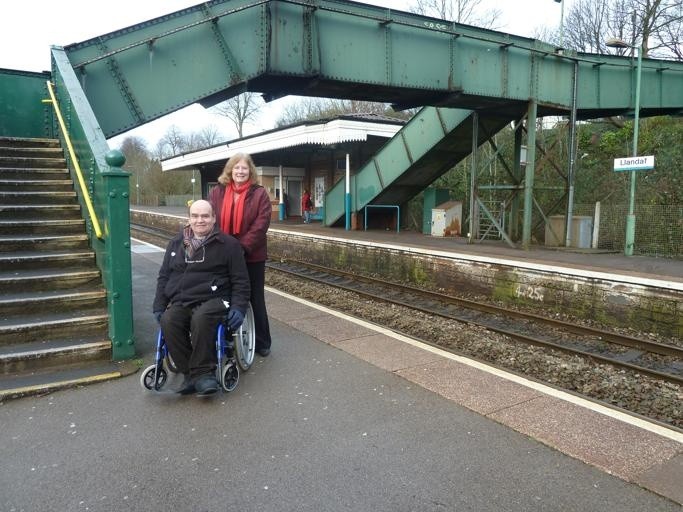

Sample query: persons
[154,199,253,395]
[283,188,290,217]
[301,188,312,223]
[212,153,272,357]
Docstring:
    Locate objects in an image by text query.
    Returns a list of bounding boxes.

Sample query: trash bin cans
[545,215,593,249]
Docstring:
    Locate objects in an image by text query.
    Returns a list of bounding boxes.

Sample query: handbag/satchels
[284,194,290,209]
[308,200,312,208]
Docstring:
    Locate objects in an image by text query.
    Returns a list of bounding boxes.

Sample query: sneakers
[195,378,218,394]
[259,350,269,357]
[175,383,193,394]
[303,220,311,224]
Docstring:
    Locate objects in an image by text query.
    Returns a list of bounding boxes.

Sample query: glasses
[185,245,206,264]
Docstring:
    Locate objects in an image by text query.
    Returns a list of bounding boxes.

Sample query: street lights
[604,37,655,257]
[553,0,564,48]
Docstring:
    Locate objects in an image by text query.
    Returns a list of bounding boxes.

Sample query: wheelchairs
[139,294,256,396]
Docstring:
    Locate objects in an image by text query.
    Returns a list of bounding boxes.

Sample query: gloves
[153,311,164,324]
[228,308,244,331]
